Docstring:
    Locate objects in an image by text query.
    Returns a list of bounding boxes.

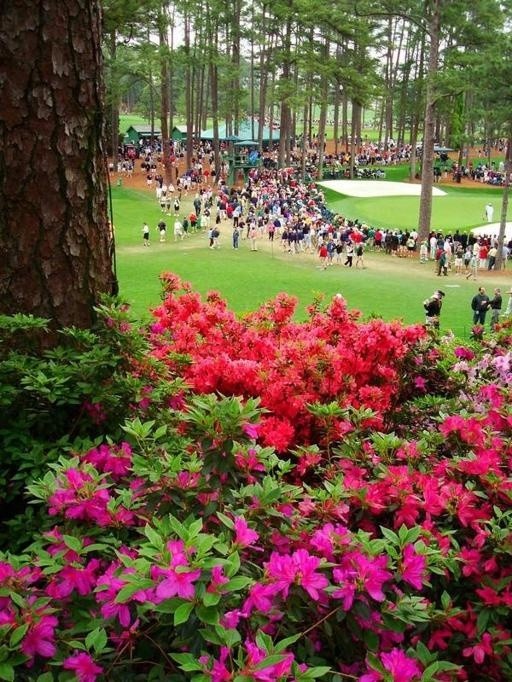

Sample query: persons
[481,288,502,333]
[504,287,512,314]
[471,287,489,334]
[423,289,445,330]
[104,112,512,282]
